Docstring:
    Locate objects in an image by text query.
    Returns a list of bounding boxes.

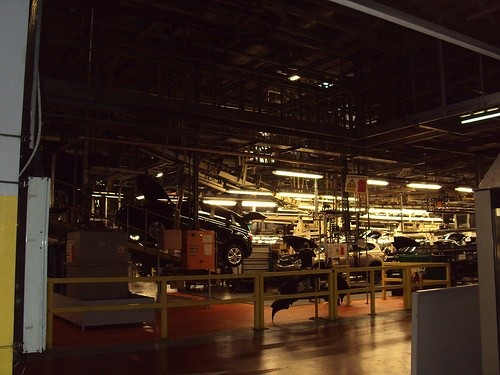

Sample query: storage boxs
[65,229,131,263]
[64,263,135,301]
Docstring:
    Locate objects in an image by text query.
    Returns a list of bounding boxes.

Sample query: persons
[299,242,316,269]
[48,207,72,263]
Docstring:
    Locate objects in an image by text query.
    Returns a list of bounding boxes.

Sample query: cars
[240,212,479,302]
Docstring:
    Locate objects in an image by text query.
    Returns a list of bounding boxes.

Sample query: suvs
[114,173,253,269]
